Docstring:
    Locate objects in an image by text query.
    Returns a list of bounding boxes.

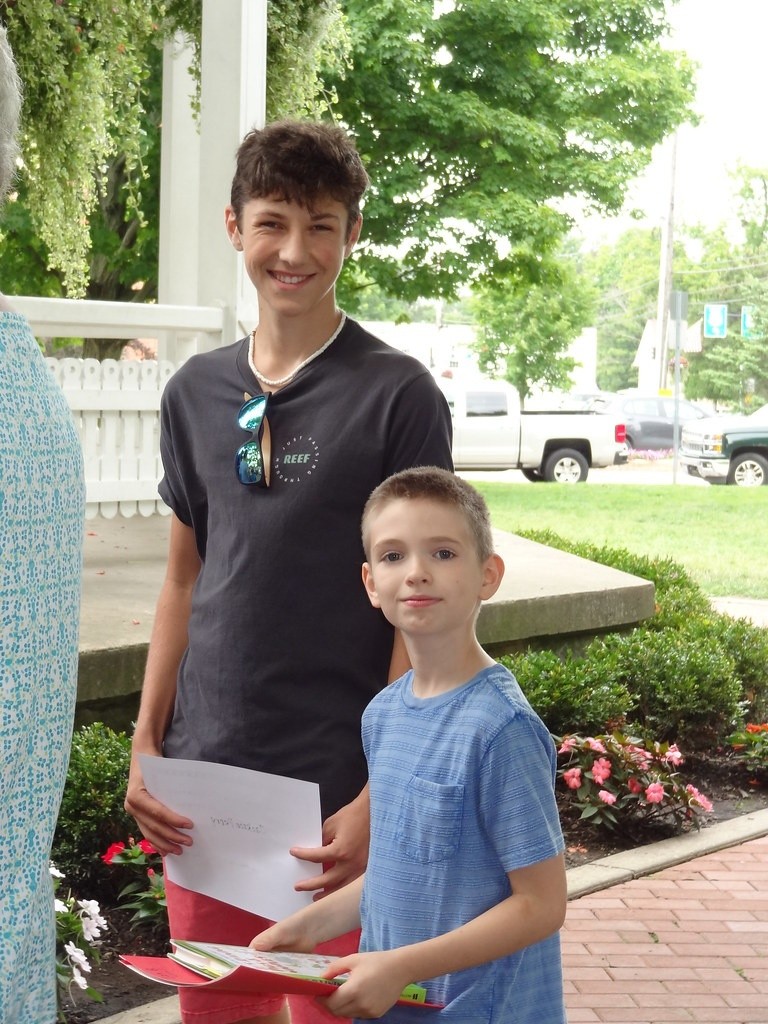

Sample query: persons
[245,465,568,1024]
[123,123,453,1024]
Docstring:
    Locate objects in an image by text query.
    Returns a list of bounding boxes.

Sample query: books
[119,934,440,1011]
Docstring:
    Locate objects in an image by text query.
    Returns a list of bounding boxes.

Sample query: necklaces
[248,307,347,385]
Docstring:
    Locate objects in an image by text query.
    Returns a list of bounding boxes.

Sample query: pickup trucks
[429,362,628,484]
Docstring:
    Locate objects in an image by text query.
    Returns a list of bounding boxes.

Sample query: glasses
[232,390,274,492]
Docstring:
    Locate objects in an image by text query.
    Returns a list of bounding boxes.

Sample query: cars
[598,395,711,452]
[677,402,768,486]
